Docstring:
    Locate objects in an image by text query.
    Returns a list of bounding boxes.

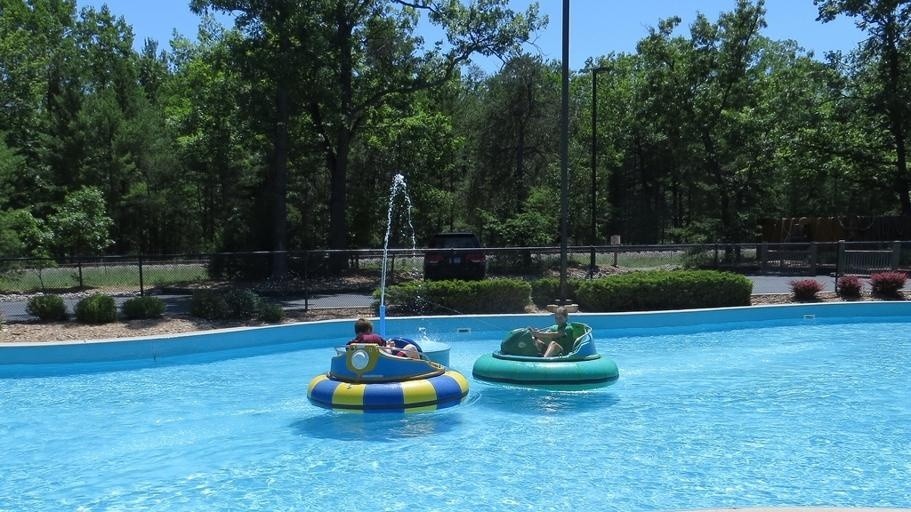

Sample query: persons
[532,307,573,357]
[346,318,420,360]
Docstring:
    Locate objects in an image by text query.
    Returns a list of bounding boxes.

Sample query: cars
[424,232,486,280]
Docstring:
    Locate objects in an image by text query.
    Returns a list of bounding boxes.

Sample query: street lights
[591,66,611,266]
